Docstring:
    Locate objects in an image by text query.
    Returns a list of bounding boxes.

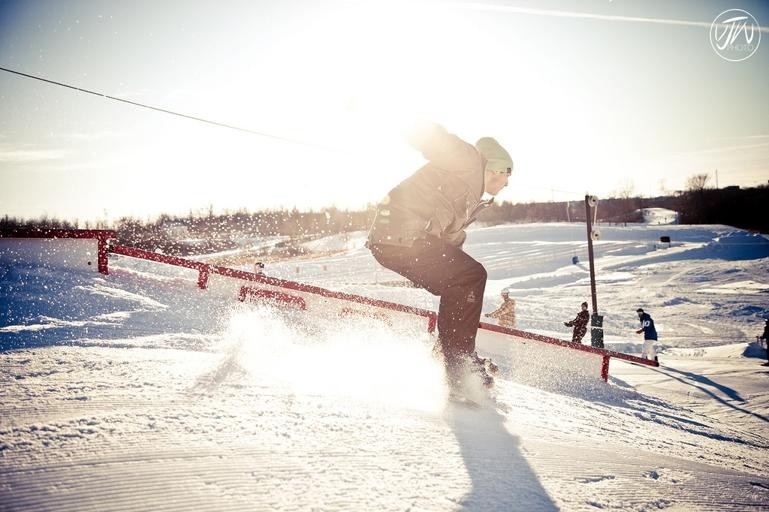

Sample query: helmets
[501,288,509,295]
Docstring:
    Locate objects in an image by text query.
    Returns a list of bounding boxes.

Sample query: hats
[474,138,513,173]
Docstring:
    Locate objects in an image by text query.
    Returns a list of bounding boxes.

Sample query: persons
[365,116,513,385]
[485,288,516,328]
[758,311,769,366]
[636,309,657,360]
[564,302,589,343]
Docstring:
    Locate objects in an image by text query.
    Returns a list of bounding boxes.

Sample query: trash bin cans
[660,236,670,249]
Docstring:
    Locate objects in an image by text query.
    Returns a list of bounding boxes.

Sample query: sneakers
[446,352,499,387]
